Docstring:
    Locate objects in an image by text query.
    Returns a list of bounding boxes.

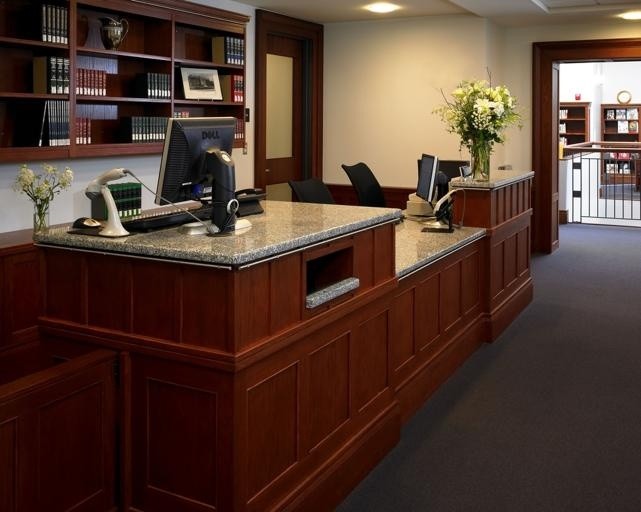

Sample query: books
[559,109,569,145]
[76,67,107,96]
[32,56,69,94]
[76,116,92,145]
[218,75,243,102]
[146,72,171,99]
[41,3,69,45]
[602,153,631,175]
[91,182,142,220]
[131,115,169,144]
[36,100,70,147]
[174,111,190,118]
[211,36,244,66]
[606,108,639,134]
[234,119,244,139]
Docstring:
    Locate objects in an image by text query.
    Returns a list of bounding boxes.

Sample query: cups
[98,16,129,51]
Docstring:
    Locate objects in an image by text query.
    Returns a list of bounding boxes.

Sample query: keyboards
[121,204,213,233]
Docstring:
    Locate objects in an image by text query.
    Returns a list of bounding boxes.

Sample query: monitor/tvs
[155,116,252,235]
[459,166,471,177]
[416,153,449,226]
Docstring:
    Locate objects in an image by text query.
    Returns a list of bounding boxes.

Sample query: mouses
[72,216,101,229]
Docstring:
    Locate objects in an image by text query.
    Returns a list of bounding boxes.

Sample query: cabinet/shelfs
[558,101,590,148]
[0,0,251,164]
[601,104,641,184]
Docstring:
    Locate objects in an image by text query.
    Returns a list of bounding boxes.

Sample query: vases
[469,145,491,180]
[31,204,49,237]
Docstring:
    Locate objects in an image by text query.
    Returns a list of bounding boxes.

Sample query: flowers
[429,72,526,177]
[14,160,74,234]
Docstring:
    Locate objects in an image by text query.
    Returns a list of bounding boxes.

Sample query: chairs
[289,178,336,204]
[342,162,387,207]
